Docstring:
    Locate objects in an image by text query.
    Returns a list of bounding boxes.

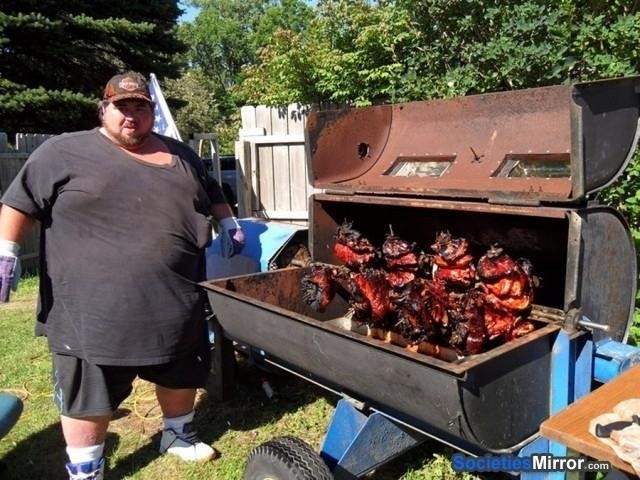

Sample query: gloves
[220,216,247,259]
[0,239,23,303]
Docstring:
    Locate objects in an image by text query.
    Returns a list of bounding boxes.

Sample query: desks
[538,361,640,480]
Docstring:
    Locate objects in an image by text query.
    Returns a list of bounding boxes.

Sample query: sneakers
[66,457,107,480]
[159,421,217,462]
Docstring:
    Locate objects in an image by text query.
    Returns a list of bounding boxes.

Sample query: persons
[0,68,246,480]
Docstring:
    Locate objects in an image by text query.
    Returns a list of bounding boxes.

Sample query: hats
[102,70,153,103]
